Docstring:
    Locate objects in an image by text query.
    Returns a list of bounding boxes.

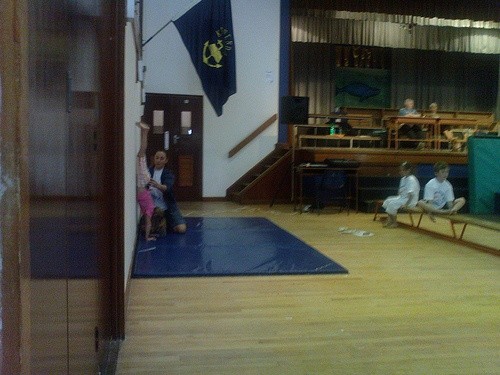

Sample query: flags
[171,0,237,116]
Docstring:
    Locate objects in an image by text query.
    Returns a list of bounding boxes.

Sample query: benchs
[373,199,500,241]
[293,107,499,149]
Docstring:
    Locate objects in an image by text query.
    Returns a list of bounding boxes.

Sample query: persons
[324,104,353,135]
[136,122,186,242]
[418,161,465,223]
[382,161,420,229]
[397,99,458,150]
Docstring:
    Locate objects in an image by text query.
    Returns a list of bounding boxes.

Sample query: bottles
[330,124,336,136]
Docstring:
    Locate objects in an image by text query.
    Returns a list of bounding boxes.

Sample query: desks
[294,166,360,213]
[386,116,478,150]
